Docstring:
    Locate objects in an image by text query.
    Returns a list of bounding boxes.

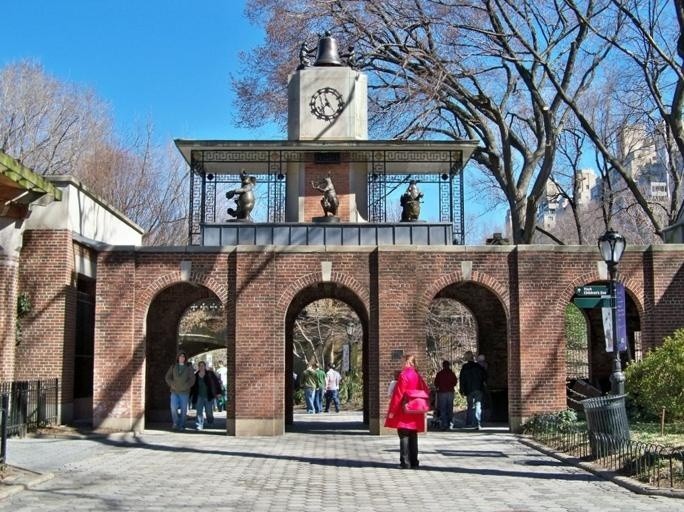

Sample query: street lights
[597,227,628,396]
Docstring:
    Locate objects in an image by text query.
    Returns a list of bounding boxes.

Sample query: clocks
[310,88,345,121]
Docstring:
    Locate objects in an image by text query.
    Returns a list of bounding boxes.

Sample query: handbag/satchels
[208,370,222,398]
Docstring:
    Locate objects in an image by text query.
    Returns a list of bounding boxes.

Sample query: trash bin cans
[579,392,629,456]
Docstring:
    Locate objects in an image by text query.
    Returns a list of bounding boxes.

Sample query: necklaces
[384,355,430,469]
[388,371,401,399]
[291,360,342,414]
[166,353,228,430]
[459,351,487,430]
[433,361,457,430]
[476,354,488,369]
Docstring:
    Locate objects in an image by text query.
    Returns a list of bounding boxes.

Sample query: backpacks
[402,388,431,413]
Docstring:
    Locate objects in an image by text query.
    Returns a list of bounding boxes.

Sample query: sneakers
[396,460,419,470]
[306,408,342,416]
[437,425,486,432]
[171,420,217,433]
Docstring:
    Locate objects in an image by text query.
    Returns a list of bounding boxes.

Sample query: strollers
[430,389,442,429]
